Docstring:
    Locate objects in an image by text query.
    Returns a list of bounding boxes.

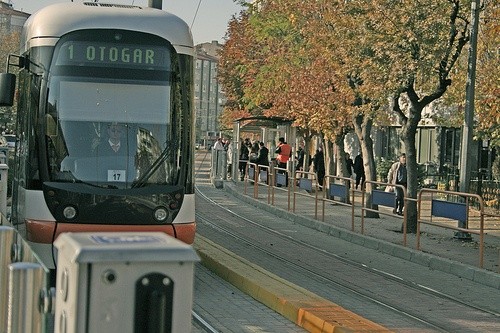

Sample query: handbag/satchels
[384,185,394,193]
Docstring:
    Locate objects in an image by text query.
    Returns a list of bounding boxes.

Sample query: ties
[112,145,120,153]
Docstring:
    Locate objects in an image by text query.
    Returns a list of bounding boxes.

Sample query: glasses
[110,128,121,133]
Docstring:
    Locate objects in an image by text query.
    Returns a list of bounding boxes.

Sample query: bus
[0,0,196,280]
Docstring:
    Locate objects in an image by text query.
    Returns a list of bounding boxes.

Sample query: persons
[296,148,365,191]
[214,137,292,187]
[386,153,407,215]
[96,121,126,156]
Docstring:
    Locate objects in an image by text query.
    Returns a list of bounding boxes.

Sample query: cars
[2,135,16,152]
